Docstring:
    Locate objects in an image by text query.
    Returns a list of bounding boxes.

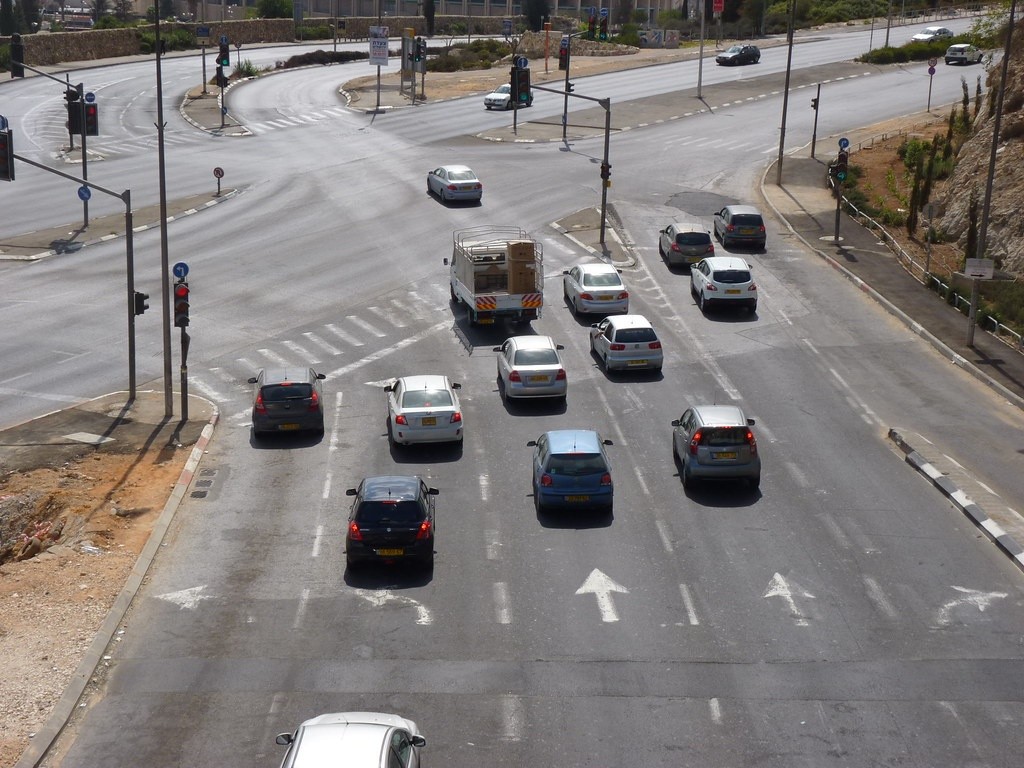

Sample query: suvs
[688,256,757,313]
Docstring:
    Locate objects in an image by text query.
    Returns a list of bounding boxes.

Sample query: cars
[426,164,482,203]
[945,43,984,65]
[911,26,954,46]
[713,205,767,249]
[384,374,464,447]
[247,366,327,437]
[671,404,761,490]
[275,711,426,768]
[492,334,568,403]
[562,264,628,318]
[715,43,761,67]
[527,429,614,514]
[658,223,715,265]
[346,475,439,571]
[588,315,664,373]
[484,83,533,111]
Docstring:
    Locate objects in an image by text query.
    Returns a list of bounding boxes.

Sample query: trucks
[443,224,545,328]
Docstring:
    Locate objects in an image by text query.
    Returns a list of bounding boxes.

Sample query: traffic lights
[224,76,230,87]
[587,14,607,40]
[135,292,151,315]
[219,43,230,66]
[509,67,531,105]
[0,130,15,181]
[64,102,82,135]
[836,151,848,183]
[174,283,190,327]
[415,43,425,61]
[84,103,98,136]
[810,98,818,109]
[559,48,567,70]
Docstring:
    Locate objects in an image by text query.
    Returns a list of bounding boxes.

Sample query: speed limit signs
[928,57,937,67]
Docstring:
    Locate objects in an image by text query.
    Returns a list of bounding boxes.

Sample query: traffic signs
[963,257,994,281]
